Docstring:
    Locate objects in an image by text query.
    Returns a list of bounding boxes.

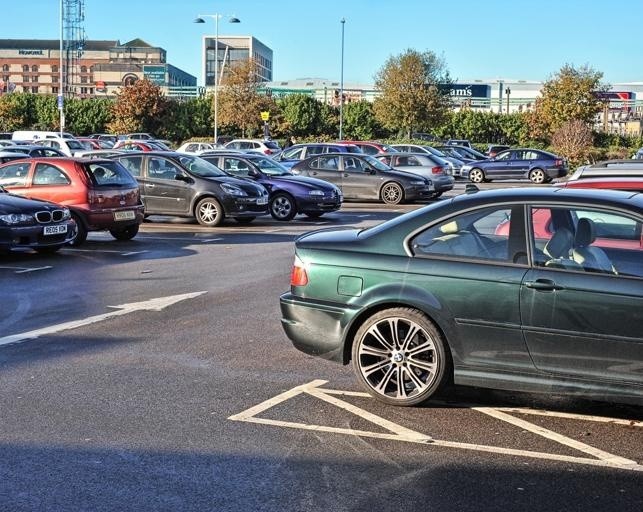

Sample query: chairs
[544,217,614,273]
[225,161,249,175]
[343,159,356,171]
[93,167,106,180]
[397,157,407,166]
[313,160,329,167]
[149,160,176,178]
[416,227,488,258]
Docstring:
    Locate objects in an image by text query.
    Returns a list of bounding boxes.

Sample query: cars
[371,151,456,200]
[197,151,344,221]
[287,152,438,205]
[0,131,174,164]
[392,140,513,177]
[278,181,643,408]
[0,156,146,245]
[458,146,569,183]
[104,151,270,226]
[493,158,643,253]
[0,184,78,254]
[175,141,224,152]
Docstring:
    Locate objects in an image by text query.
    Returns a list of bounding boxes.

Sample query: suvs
[269,143,363,170]
[222,138,283,159]
[336,140,398,166]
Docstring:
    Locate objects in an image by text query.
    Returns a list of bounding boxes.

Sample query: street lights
[337,17,347,140]
[190,12,241,147]
